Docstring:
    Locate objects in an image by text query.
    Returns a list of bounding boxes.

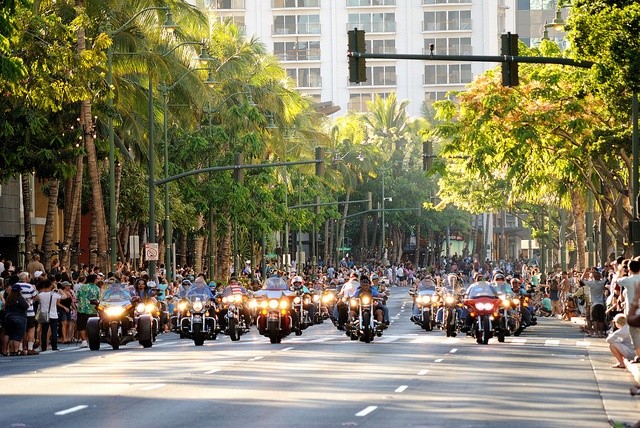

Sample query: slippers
[611,363,626,368]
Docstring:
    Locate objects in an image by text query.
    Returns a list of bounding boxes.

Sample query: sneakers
[592,333,599,337]
[585,333,591,336]
[79,339,88,348]
[344,323,356,331]
[521,324,525,328]
[377,329,382,337]
[608,418,638,427]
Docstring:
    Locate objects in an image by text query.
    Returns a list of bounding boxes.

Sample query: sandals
[10,350,15,355]
[14,349,24,355]
[1,351,10,357]
[635,354,640,362]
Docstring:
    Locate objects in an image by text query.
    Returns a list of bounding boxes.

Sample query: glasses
[183,282,189,285]
[209,285,215,287]
[195,281,204,283]
[507,278,511,281]
[231,281,236,282]
[512,281,517,283]
[138,282,144,284]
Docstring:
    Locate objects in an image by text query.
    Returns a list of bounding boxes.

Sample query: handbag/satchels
[37,291,53,323]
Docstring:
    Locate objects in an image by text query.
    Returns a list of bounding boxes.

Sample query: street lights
[202,91,258,280]
[148,39,214,287]
[157,65,221,282]
[234,111,279,276]
[98,7,179,274]
[381,196,392,257]
[544,4,638,219]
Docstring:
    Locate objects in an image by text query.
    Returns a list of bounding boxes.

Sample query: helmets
[495,274,504,281]
[495,269,503,275]
[292,275,302,288]
[253,278,259,285]
[147,281,156,288]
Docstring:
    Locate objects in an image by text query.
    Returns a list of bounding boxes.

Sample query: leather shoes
[52,347,59,351]
[27,348,39,355]
[20,349,25,356]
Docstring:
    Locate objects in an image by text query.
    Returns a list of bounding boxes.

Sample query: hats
[173,280,179,285]
[209,281,216,285]
[61,280,73,287]
[33,270,43,278]
[11,285,22,290]
[362,278,369,284]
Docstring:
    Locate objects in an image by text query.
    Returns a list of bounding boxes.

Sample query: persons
[38,280,67,350]
[76,275,102,348]
[0,279,5,355]
[16,271,39,355]
[410,252,537,326]
[615,261,640,364]
[606,314,636,368]
[0,254,105,284]
[534,258,618,336]
[5,284,30,356]
[57,281,77,344]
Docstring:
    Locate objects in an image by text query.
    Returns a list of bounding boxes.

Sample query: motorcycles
[219,286,250,340]
[409,281,537,344]
[291,288,337,335]
[248,276,301,344]
[168,277,221,345]
[87,284,158,349]
[343,291,388,343]
